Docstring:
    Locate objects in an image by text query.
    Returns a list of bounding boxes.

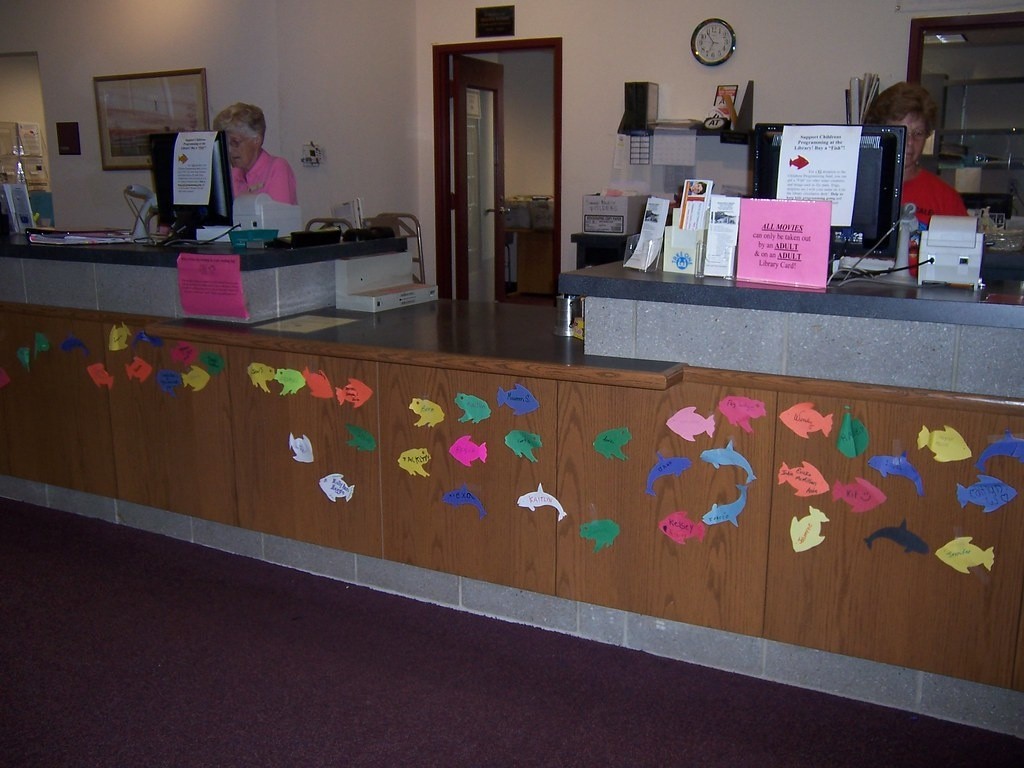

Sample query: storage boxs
[503,197,533,228]
[530,197,554,229]
[583,193,650,235]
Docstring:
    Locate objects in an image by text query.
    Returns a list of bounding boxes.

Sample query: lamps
[128,183,158,240]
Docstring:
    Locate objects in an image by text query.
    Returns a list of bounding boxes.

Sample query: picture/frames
[92,66,210,172]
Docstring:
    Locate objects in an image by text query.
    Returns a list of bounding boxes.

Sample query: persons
[862,81,968,282]
[688,179,707,196]
[212,102,297,206]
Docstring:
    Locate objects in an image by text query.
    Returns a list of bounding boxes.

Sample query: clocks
[690,17,737,66]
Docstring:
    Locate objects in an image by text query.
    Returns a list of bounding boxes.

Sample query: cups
[552,296,581,337]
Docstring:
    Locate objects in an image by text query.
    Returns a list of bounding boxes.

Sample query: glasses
[228,138,244,148]
[906,128,930,141]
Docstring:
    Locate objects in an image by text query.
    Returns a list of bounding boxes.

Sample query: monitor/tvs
[148,130,232,239]
[752,122,907,274]
